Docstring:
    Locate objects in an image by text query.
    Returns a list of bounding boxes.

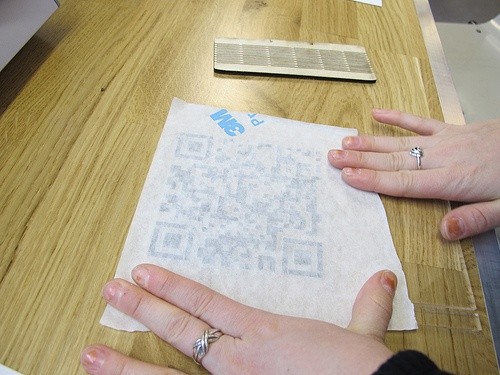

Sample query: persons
[82,107,500,375]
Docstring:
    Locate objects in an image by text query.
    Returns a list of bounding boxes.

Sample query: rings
[409,146,425,170]
[191,327,225,369]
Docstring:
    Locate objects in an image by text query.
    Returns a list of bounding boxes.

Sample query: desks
[1,0,500,374]
